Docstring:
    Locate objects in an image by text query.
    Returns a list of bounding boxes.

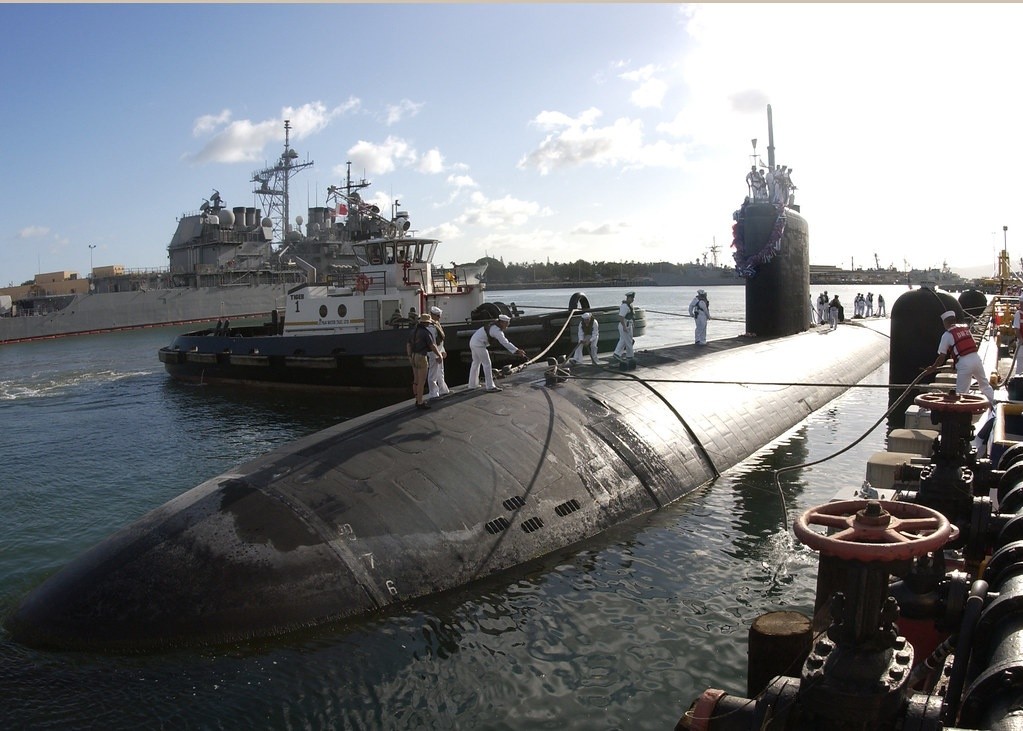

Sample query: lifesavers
[355,274,369,291]
[569,293,591,311]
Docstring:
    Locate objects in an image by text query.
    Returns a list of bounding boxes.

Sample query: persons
[1011,292,1023,375]
[746,164,794,204]
[398,250,406,262]
[407,314,442,410]
[611,291,636,365]
[924,310,995,400]
[569,312,600,367]
[853,291,874,317]
[427,306,454,401]
[467,313,527,392]
[688,289,712,346]
[877,293,886,317]
[809,291,841,330]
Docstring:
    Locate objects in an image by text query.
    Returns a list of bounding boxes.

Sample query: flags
[327,203,348,216]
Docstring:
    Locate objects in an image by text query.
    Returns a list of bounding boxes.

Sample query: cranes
[708,245,723,266]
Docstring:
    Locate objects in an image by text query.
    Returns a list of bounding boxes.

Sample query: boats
[157,161,647,405]
[0,118,487,342]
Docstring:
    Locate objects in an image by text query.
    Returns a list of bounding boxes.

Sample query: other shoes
[626,357,636,361]
[613,354,620,360]
[487,387,503,392]
[468,385,481,390]
[430,396,443,400]
[415,402,431,409]
[695,341,700,345]
[440,390,454,396]
[699,342,707,346]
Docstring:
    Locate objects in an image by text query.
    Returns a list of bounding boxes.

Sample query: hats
[582,312,592,320]
[697,290,704,294]
[624,291,636,296]
[418,314,431,322]
[499,314,512,322]
[431,306,443,317]
[940,311,955,320]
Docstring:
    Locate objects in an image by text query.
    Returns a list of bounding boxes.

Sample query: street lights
[88,245,97,269]
